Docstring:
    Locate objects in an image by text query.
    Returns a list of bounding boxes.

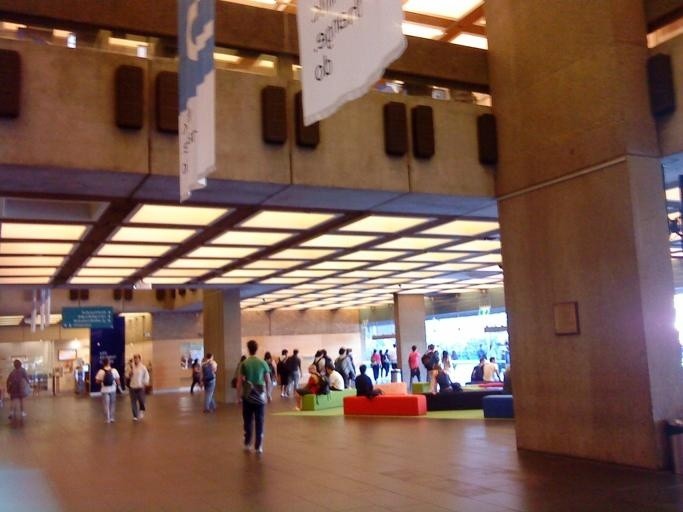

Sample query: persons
[202,353,218,414]
[126,353,150,422]
[190,359,204,393]
[94,358,121,423]
[236,339,273,454]
[232,355,247,403]
[262,344,505,397]
[3,359,33,418]
[187,358,193,368]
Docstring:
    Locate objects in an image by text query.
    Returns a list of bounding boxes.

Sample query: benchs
[483,395,513,418]
[343,395,426,416]
[422,389,502,411]
[411,381,429,394]
[362,382,407,395]
[301,389,355,410]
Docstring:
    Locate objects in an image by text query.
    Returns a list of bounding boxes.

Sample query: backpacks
[266,357,347,395]
[102,368,113,386]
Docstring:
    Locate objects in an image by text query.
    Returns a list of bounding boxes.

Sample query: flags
[295,0,409,129]
[176,1,218,206]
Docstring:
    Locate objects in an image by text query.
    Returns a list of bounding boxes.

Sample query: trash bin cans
[664,418,683,475]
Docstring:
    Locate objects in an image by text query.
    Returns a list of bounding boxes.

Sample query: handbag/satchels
[126,363,132,387]
[451,383,462,392]
[232,378,237,388]
[245,383,266,405]
[202,363,213,382]
